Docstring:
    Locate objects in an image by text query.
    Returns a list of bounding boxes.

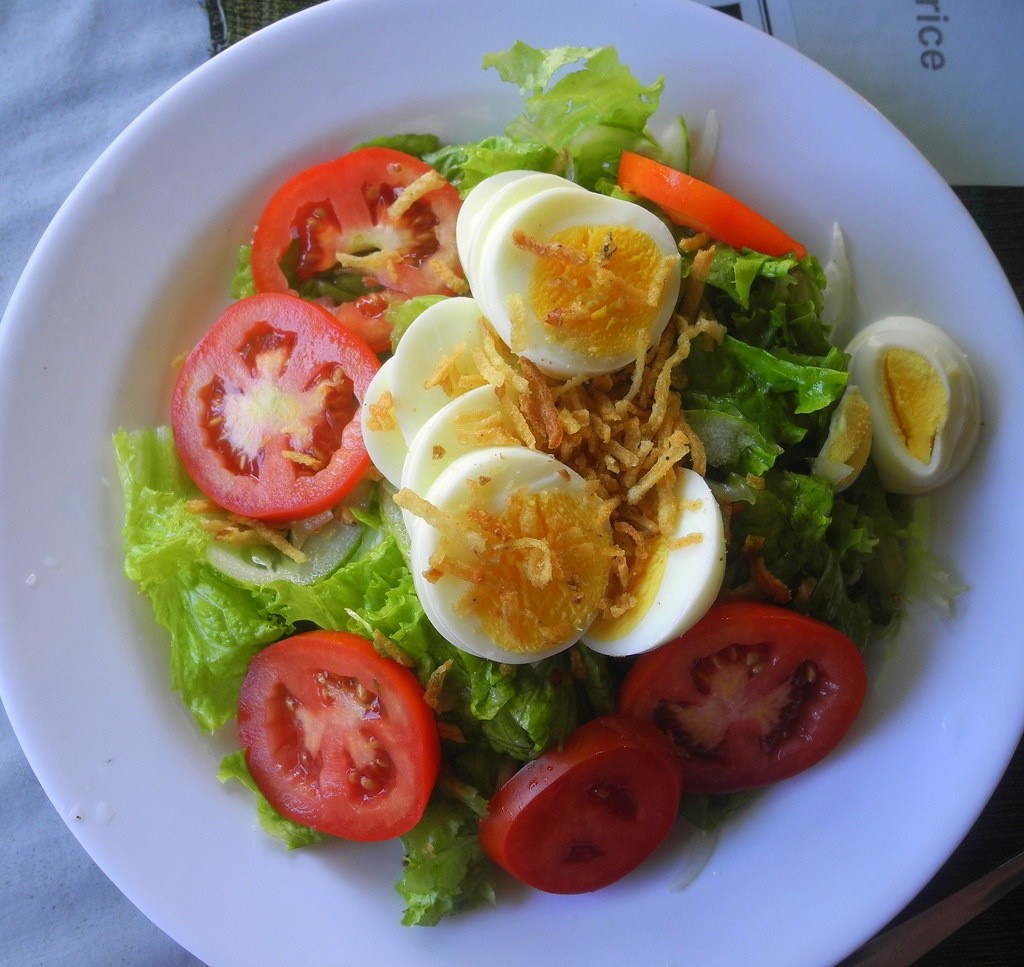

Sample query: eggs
[360,355,411,489]
[390,298,505,447]
[411,444,613,666]
[577,466,727,657]
[813,385,873,493]
[476,187,682,380]
[466,172,587,315]
[843,315,984,499]
[456,170,556,280]
[401,383,511,537]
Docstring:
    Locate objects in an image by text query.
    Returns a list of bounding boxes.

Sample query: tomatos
[450,713,681,895]
[250,148,468,356]
[235,631,441,842]
[617,603,868,795]
[614,151,804,260]
[170,293,384,522]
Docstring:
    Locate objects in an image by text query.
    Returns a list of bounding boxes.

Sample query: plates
[0,1,1024,967]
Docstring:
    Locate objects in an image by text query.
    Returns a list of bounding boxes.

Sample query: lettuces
[110,43,917,929]
